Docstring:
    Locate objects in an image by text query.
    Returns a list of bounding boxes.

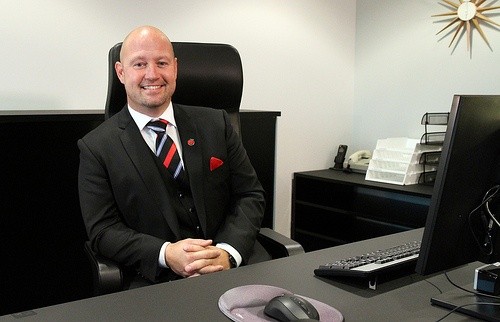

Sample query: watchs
[227,251,237,268]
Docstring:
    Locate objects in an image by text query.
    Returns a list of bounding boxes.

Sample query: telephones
[334,145,348,168]
[343,150,372,174]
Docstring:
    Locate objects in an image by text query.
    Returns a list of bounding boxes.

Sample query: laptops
[313,94,500,286]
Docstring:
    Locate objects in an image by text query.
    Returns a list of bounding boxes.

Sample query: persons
[78,25,271,291]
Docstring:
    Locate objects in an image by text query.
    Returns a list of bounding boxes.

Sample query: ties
[146,119,183,181]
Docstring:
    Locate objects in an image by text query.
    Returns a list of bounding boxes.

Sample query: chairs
[81,41,306,299]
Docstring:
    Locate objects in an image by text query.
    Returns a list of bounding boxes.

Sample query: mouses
[265,295,319,322]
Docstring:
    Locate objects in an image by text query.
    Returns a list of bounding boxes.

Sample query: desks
[0,108,282,315]
[0,227,484,322]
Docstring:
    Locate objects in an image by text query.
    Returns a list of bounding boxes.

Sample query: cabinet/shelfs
[364,136,443,186]
[419,112,450,186]
[289,168,435,253]
